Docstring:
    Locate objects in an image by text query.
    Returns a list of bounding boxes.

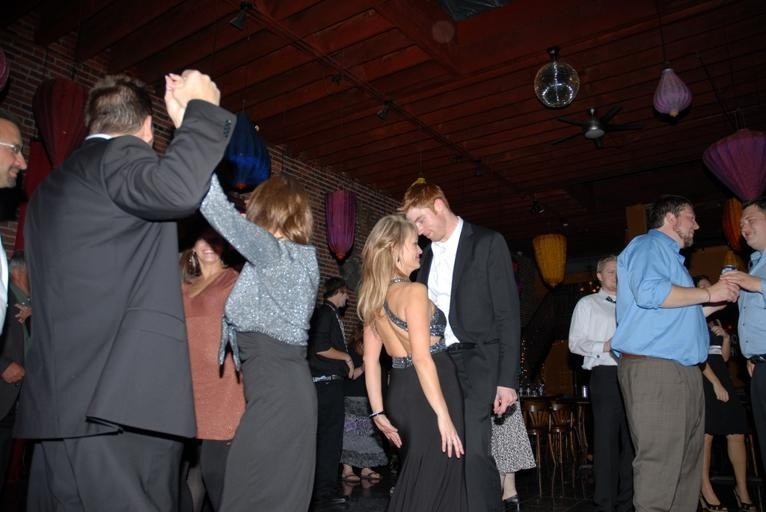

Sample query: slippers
[359,471,381,479]
[340,474,361,482]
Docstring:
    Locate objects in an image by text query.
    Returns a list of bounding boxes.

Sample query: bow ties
[606,295,616,303]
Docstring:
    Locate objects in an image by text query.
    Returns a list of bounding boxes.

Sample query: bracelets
[703,286,711,302]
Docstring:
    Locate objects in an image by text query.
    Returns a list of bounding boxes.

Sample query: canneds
[720,265,736,275]
[581,385,589,400]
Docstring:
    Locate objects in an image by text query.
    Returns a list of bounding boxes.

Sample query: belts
[751,355,766,363]
[312,373,343,382]
[621,353,646,359]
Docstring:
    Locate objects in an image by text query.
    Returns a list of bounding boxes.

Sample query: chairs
[521,396,590,465]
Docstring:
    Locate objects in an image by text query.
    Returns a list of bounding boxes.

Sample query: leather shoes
[311,496,345,505]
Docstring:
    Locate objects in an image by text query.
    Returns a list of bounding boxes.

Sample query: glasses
[0,142,20,153]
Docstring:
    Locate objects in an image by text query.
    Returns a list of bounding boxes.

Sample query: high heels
[732,486,757,512]
[699,489,728,512]
[503,494,521,511]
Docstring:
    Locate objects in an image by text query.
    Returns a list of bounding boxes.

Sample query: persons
[568,254,634,512]
[719,199,766,512]
[307,177,535,512]
[692,275,757,512]
[610,194,740,512]
[1,71,320,512]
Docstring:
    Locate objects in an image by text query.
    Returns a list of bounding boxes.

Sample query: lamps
[530,1,766,289]
[0,0,358,265]
